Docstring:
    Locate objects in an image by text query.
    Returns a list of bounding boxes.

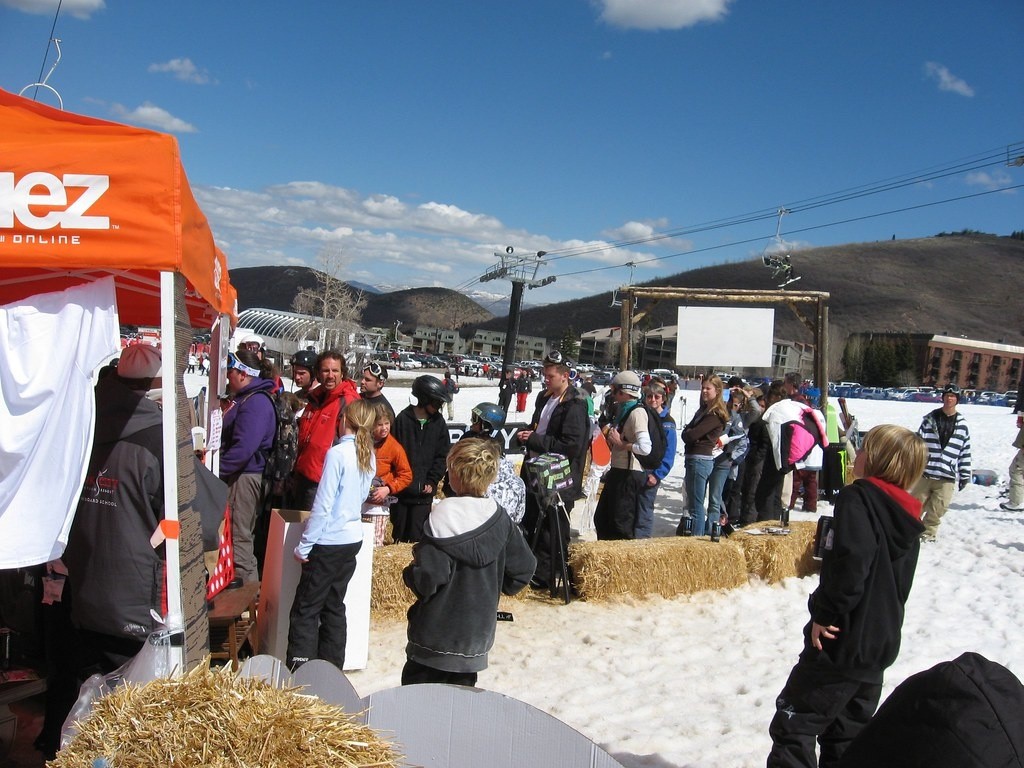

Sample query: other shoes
[921,536,937,543]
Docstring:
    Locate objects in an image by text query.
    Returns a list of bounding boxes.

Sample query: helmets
[239,335,267,352]
[471,402,506,431]
[289,350,319,380]
[410,375,453,403]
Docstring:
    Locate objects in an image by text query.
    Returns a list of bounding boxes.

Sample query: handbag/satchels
[58,628,172,750]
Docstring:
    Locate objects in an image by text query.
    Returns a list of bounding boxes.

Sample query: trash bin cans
[502,365,514,379]
[823,443,847,500]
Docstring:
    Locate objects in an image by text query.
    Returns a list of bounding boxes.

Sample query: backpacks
[618,403,666,469]
[262,397,299,497]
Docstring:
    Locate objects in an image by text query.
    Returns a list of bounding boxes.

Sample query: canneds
[0,628,12,671]
[711,521,721,542]
[683,516,692,536]
[779,507,789,528]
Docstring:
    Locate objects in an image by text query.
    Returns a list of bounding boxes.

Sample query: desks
[207,581,260,671]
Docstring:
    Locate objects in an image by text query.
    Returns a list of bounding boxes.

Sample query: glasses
[362,361,387,382]
[545,350,563,364]
[427,395,442,410]
[733,403,742,409]
[472,411,482,425]
[663,387,666,391]
[943,385,960,394]
[227,352,264,378]
[645,393,662,399]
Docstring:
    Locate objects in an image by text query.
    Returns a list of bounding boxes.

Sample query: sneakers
[1000,500,1024,511]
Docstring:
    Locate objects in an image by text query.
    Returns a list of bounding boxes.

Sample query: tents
[0,84,240,684]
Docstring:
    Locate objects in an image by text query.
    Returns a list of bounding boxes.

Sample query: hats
[613,370,641,397]
[943,382,959,405]
[117,343,162,402]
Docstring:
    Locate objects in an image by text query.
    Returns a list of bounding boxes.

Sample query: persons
[286,398,377,677]
[400,437,538,688]
[910,383,972,545]
[76,335,830,594]
[766,423,928,768]
[55,343,164,670]
[999,374,1024,513]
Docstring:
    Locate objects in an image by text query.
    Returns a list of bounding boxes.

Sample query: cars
[717,372,772,388]
[803,379,1017,407]
[120,333,210,344]
[370,352,503,377]
[589,368,680,386]
[515,360,595,378]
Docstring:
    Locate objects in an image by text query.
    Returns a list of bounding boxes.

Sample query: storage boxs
[257,508,375,669]
